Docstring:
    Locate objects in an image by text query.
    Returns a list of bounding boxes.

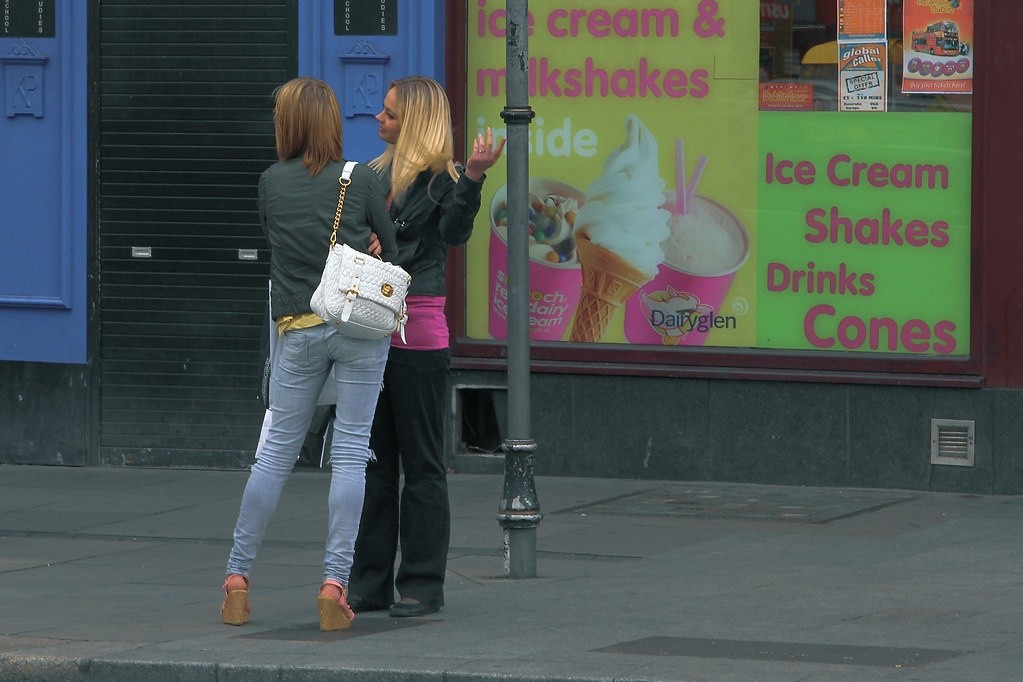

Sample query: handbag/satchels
[309,161,412,345]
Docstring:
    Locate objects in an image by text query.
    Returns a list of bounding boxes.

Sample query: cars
[759,37,972,113]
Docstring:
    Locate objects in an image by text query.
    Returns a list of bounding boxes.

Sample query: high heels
[219,572,250,625]
[318,579,355,631]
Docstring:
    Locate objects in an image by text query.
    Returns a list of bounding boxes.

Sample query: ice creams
[496,189,586,266]
[571,112,672,345]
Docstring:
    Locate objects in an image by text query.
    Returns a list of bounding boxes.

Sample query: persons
[348,76,506,619]
[220,78,410,632]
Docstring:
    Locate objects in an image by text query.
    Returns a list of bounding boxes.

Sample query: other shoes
[346,595,391,613]
[390,596,439,616]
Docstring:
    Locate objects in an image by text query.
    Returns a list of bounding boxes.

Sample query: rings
[479,150,485,153]
